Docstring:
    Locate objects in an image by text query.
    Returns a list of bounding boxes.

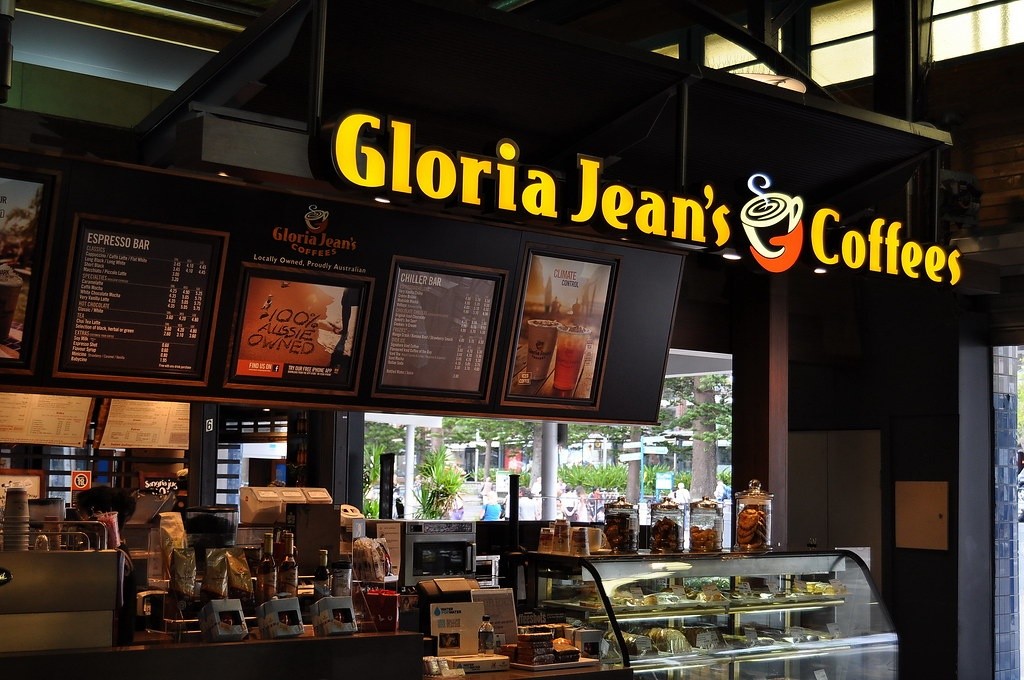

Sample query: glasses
[77,508,91,520]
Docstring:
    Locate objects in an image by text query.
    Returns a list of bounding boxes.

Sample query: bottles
[332,560,352,596]
[314,549,331,604]
[478,615,496,657]
[257,532,277,603]
[279,533,299,598]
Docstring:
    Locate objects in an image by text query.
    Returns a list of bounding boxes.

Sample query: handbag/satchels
[480,504,487,520]
[450,499,464,519]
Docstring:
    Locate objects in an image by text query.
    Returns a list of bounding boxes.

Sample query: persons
[478,478,604,521]
[713,479,726,500]
[668,483,691,504]
[76,485,138,647]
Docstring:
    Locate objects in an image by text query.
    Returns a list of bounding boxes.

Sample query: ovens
[404,520,478,586]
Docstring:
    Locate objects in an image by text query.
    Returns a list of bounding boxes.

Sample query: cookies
[736,503,768,550]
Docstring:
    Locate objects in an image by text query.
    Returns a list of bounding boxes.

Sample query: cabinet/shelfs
[510,549,901,680]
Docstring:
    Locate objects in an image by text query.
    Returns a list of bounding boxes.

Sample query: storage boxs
[255,597,305,639]
[310,596,359,637]
[364,593,401,632]
[439,653,511,672]
[198,598,249,643]
[566,628,602,663]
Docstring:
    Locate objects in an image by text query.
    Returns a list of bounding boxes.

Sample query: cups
[571,527,590,557]
[537,528,554,554]
[553,326,592,390]
[527,320,563,380]
[42,516,62,550]
[96,512,121,549]
[0,275,23,342]
[3,488,30,552]
[552,519,572,554]
[549,522,556,528]
[588,528,602,552]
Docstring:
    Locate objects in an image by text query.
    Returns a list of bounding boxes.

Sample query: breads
[578,581,850,656]
[500,632,580,666]
